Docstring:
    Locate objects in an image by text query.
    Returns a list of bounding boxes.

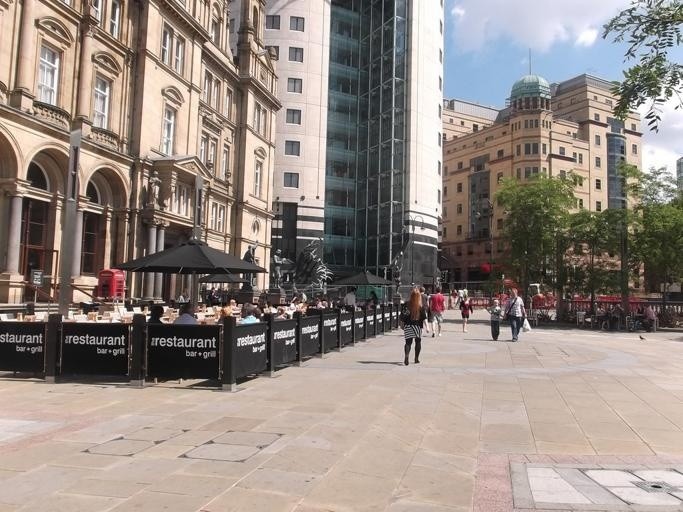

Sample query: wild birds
[639,335,645,341]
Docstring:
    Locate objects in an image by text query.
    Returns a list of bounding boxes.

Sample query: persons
[504,288,527,342]
[419,287,473,337]
[634,303,644,329]
[611,304,623,320]
[272,248,283,288]
[240,239,259,283]
[486,300,504,341]
[398,285,429,365]
[640,304,655,331]
[149,284,379,326]
[596,302,610,329]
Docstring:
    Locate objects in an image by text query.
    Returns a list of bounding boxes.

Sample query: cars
[526,283,547,297]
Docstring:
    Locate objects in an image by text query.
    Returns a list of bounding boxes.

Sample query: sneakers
[493,337,498,341]
[512,338,518,342]
[415,359,420,363]
[405,360,409,365]
[503,313,509,321]
[421,330,441,338]
[463,330,468,333]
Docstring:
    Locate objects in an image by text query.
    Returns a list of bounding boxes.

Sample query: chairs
[502,306,659,332]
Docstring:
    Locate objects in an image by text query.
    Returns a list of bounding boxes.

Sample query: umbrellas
[114,241,270,305]
[336,269,394,304]
[201,272,250,300]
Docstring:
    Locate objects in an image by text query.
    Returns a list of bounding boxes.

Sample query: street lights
[473,195,509,279]
[399,212,427,285]
